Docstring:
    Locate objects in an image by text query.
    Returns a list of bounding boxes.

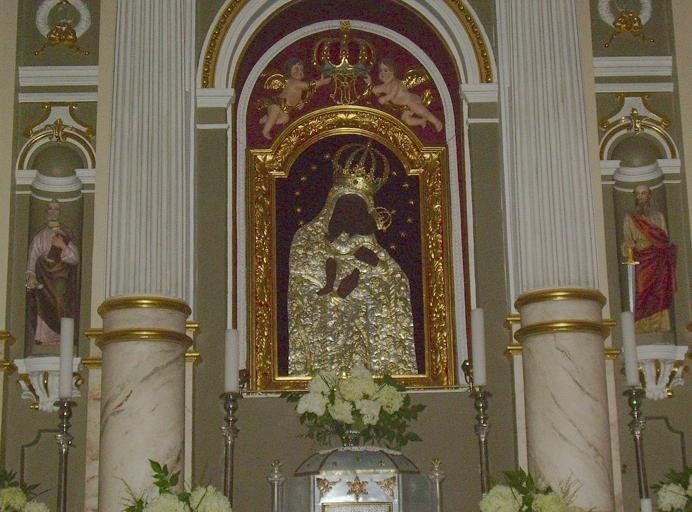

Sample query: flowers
[113,456,239,512]
[480,463,594,512]
[278,356,431,451]
[2,470,49,512]
[649,464,688,510]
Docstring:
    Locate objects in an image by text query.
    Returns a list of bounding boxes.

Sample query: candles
[470,308,487,389]
[620,313,639,389]
[58,317,77,401]
[221,330,240,394]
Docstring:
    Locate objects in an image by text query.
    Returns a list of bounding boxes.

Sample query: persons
[619,184,677,332]
[288,180,419,375]
[314,213,381,299]
[26,197,82,350]
[259,58,332,142]
[365,53,443,135]
